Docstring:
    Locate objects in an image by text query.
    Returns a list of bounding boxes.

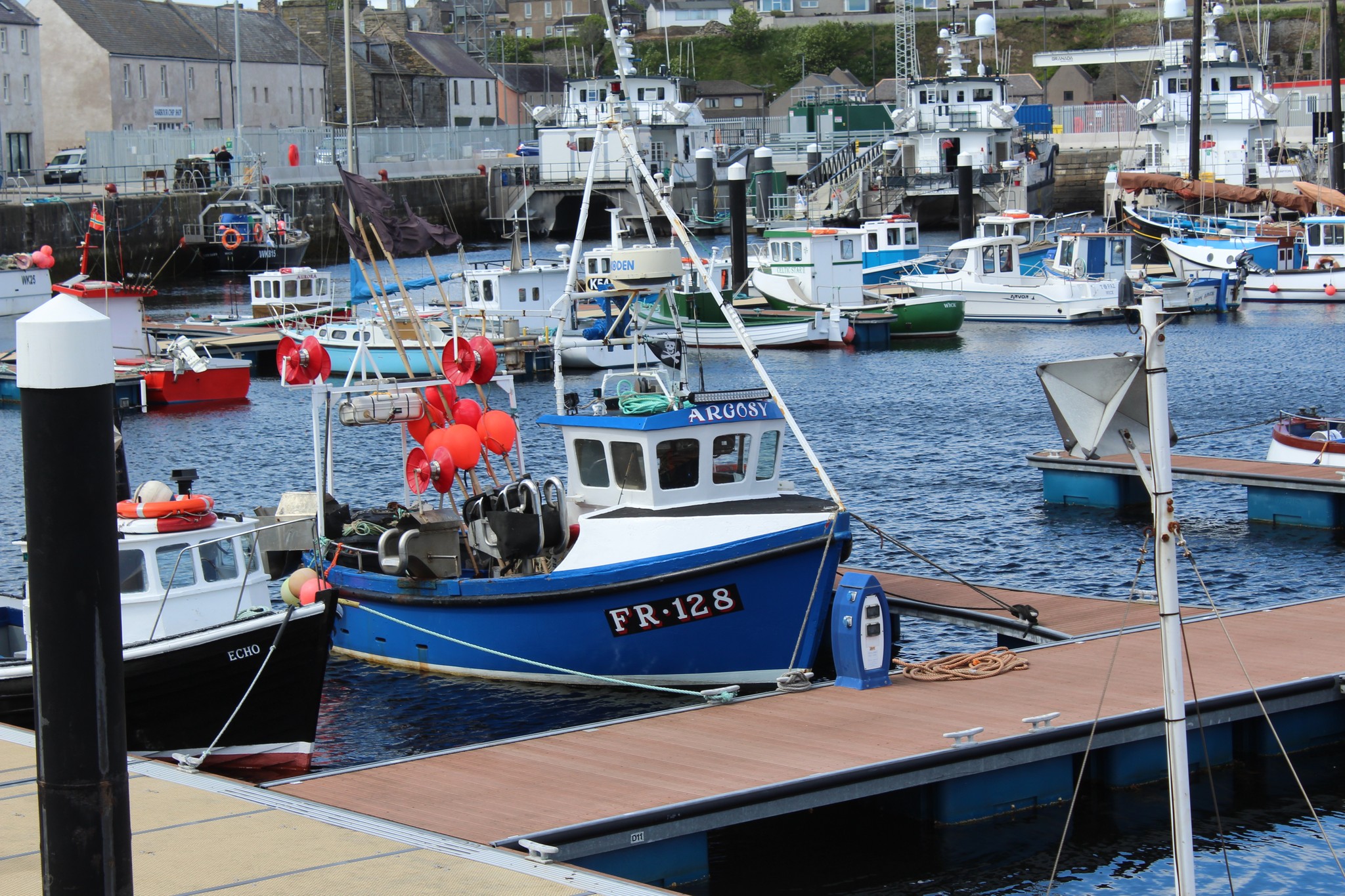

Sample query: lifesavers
[1313,256,1340,269]
[279,266,312,274]
[681,257,709,265]
[1002,209,1030,218]
[880,214,912,223]
[831,189,843,203]
[117,494,218,534]
[222,228,241,250]
[807,229,839,235]
[253,224,263,244]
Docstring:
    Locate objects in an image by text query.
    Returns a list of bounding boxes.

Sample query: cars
[425,142,504,157]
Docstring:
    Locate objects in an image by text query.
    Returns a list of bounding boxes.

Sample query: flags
[89,202,105,231]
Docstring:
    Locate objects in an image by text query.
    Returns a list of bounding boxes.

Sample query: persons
[216,145,234,191]
[209,146,220,181]
[1268,141,1287,165]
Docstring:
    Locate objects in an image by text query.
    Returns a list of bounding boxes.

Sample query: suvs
[516,140,540,157]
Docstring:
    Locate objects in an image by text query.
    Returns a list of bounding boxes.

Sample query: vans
[313,136,374,166]
[43,146,88,184]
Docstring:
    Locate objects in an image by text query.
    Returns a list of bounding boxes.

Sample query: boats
[1,468,348,787]
[1266,401,1345,467]
[112,1,1345,405]
[276,99,857,685]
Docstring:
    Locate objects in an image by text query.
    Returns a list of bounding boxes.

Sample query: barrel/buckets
[1309,429,1343,440]
